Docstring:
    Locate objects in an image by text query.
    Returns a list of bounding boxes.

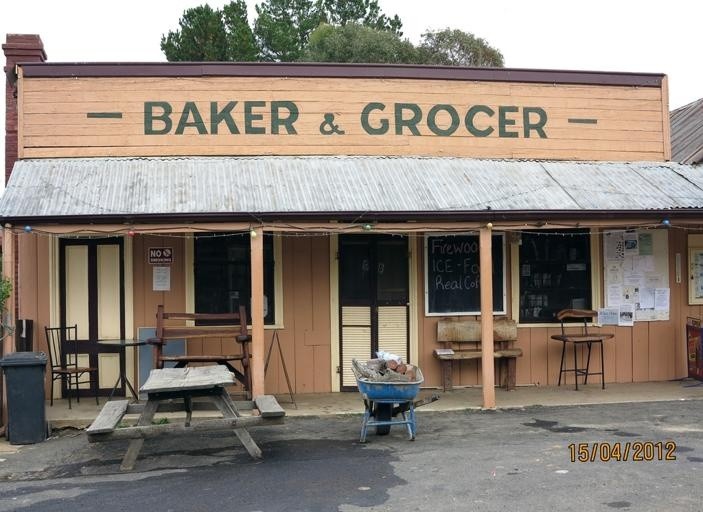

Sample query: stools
[255,394,286,418]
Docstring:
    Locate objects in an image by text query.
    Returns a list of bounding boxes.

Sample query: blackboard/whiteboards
[603,227,671,322]
[424,232,507,316]
[138,328,188,400]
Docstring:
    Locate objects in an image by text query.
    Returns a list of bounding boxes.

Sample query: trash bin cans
[0,352,48,444]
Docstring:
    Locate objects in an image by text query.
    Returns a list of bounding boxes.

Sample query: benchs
[86,399,129,435]
[432,320,523,393]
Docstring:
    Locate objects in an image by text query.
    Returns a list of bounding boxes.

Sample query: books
[434,348,454,356]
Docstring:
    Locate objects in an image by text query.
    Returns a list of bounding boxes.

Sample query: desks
[97,338,148,404]
[120,365,262,471]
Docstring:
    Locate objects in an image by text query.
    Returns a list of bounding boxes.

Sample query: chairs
[146,305,252,391]
[551,308,615,391]
[45,324,99,409]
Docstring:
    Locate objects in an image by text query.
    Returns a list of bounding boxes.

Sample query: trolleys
[349,355,425,443]
